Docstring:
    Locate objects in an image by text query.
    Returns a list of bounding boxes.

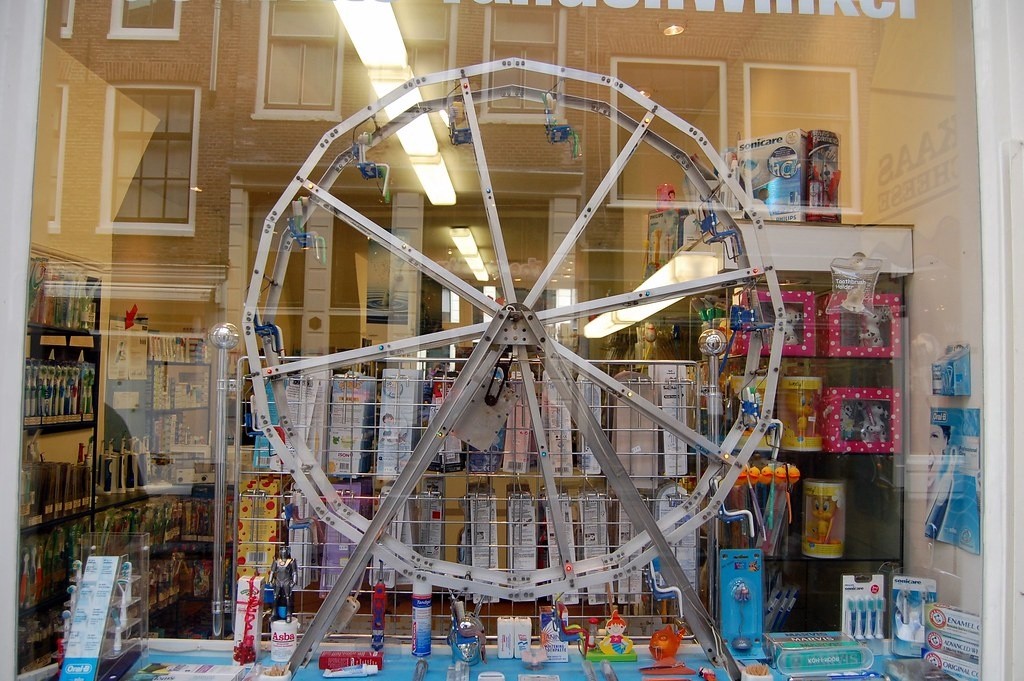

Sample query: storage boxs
[820,387,902,453]
[232,576,266,668]
[134,663,246,681]
[647,208,680,279]
[814,292,902,357]
[736,129,809,223]
[319,651,384,670]
[731,288,815,356]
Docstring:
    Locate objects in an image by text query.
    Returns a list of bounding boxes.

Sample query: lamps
[584,253,719,339]
[450,228,489,281]
[333,0,456,206]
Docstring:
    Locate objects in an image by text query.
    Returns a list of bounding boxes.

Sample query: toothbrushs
[848,595,886,641]
[921,586,928,625]
[18,496,234,609]
[902,588,909,624]
[62,558,133,657]
[23,357,95,417]
[29,256,103,331]
[23,413,212,524]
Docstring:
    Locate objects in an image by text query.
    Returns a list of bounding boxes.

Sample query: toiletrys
[323,663,378,678]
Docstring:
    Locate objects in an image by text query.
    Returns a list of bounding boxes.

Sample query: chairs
[254,79,784,659]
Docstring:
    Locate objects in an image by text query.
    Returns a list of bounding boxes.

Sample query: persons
[269,547,298,622]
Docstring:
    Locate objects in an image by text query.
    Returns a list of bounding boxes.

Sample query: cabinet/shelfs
[636,222,914,633]
[16,242,241,681]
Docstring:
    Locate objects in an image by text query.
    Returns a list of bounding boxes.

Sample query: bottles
[587,618,597,648]
